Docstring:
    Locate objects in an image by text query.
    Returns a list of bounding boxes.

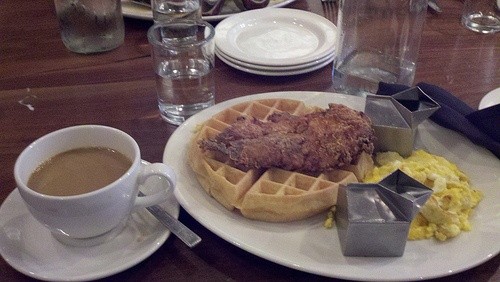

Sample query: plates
[0,157,179,281]
[108,0,298,23]
[211,7,348,76]
[165,91,500,282]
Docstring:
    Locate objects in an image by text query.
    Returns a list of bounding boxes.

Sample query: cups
[146,18,219,124]
[460,0,500,34]
[14,123,177,238]
[331,0,427,96]
[53,0,125,55]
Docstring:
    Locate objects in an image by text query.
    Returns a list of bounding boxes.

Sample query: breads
[189,97,373,222]
